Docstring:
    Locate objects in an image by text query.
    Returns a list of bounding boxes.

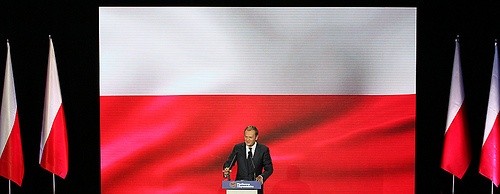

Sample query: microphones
[229,151,239,170]
[248,151,255,172]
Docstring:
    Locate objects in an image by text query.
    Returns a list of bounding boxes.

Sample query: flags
[38,37,71,179]
[441,41,472,179]
[479,43,500,189]
[0,40,24,186]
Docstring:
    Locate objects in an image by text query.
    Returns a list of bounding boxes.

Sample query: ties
[248,148,252,174]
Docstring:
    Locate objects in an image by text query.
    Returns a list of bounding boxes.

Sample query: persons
[222,126,274,194]
